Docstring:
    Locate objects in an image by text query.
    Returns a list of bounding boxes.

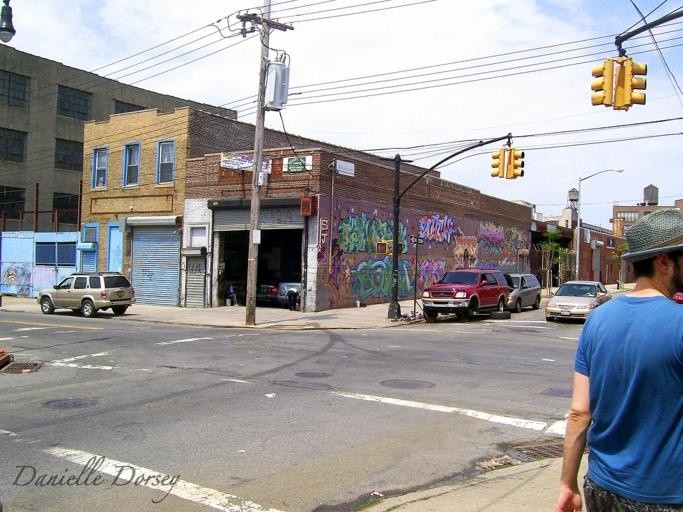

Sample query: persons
[226,283,239,307]
[555,208,683,512]
[285,287,301,311]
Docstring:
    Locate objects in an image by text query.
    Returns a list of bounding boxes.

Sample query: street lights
[575,168,624,282]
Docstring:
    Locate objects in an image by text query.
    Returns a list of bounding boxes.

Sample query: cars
[546,281,612,322]
[257,270,301,306]
[37,273,136,318]
[422,269,509,318]
[505,274,541,313]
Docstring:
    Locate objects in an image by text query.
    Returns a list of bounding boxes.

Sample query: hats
[620,208,683,262]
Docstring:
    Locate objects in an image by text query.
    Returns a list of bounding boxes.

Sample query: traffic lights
[589,56,649,113]
[376,240,393,256]
[489,144,525,179]
